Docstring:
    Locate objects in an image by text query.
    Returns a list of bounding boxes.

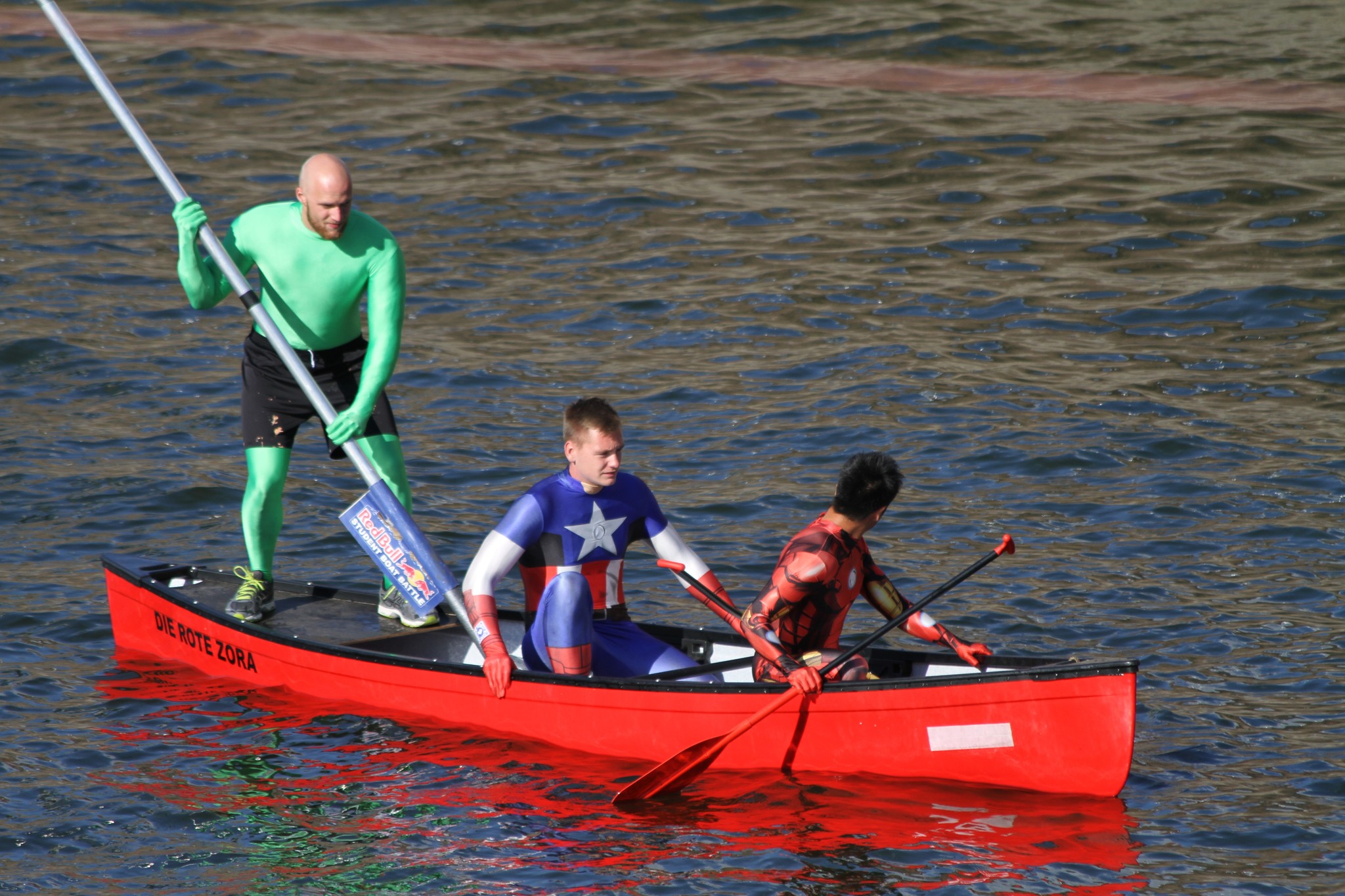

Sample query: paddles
[612,534,1016,803]
[657,558,744,618]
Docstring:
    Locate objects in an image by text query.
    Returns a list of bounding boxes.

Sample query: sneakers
[224,565,276,623]
[377,577,440,628]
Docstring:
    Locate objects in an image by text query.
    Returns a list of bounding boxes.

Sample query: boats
[98,546,1141,801]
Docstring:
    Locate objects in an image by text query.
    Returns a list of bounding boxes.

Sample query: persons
[171,155,441,629]
[463,398,749,701]
[739,451,991,698]
